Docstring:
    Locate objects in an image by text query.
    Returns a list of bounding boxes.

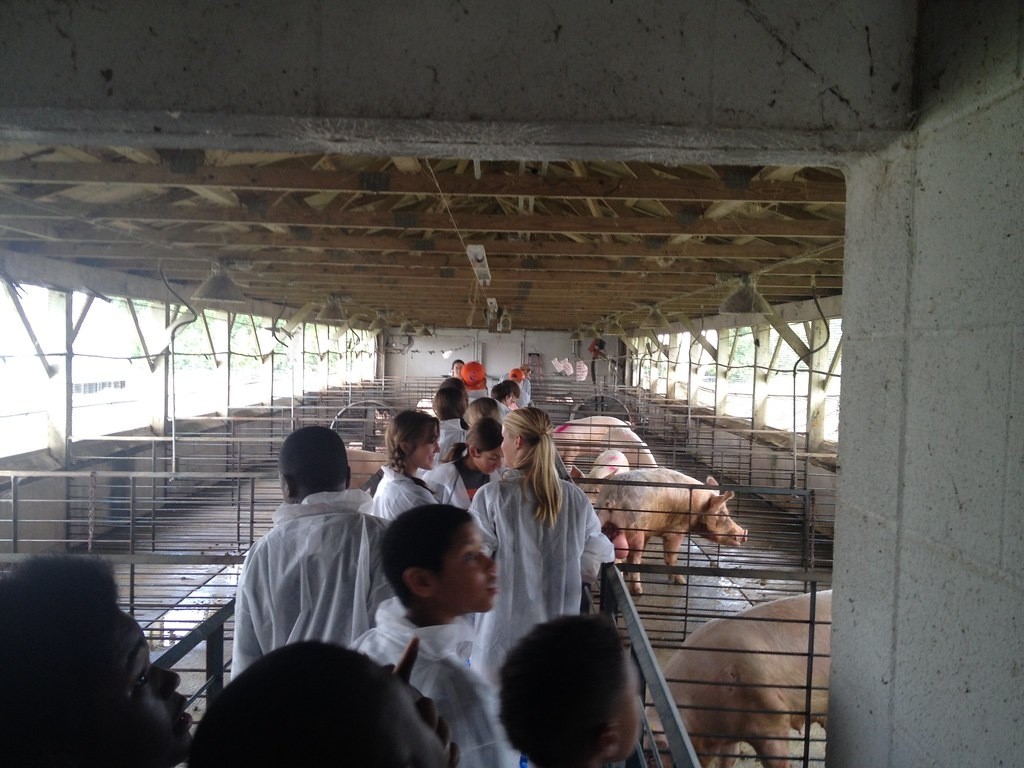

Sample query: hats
[520,363,532,370]
[510,369,524,383]
[461,361,485,386]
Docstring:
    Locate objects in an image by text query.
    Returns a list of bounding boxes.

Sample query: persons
[188,638,460,768]
[422,417,503,511]
[466,407,615,685]
[0,551,193,768]
[431,385,469,470]
[439,359,535,426]
[233,425,398,679]
[497,613,644,768]
[345,503,534,768]
[372,410,441,526]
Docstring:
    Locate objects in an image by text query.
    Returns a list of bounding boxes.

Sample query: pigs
[643,586,832,768]
[601,428,659,471]
[569,450,630,509]
[594,467,748,595]
[346,399,438,490]
[550,415,631,475]
